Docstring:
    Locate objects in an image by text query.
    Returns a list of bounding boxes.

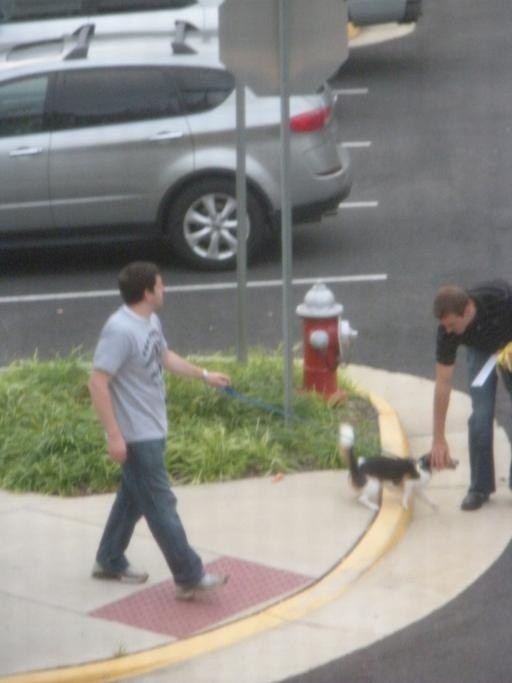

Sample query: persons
[430,277,512,511]
[90,262,234,599]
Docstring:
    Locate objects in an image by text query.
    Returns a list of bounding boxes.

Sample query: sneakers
[175,572,231,601]
[460,489,490,512]
[92,559,149,585]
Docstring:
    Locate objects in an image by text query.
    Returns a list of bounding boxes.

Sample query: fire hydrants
[295,278,358,405]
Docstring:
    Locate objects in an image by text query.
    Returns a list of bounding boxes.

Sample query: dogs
[335,421,464,515]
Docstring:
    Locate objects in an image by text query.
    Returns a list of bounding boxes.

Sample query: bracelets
[202,367,209,382]
